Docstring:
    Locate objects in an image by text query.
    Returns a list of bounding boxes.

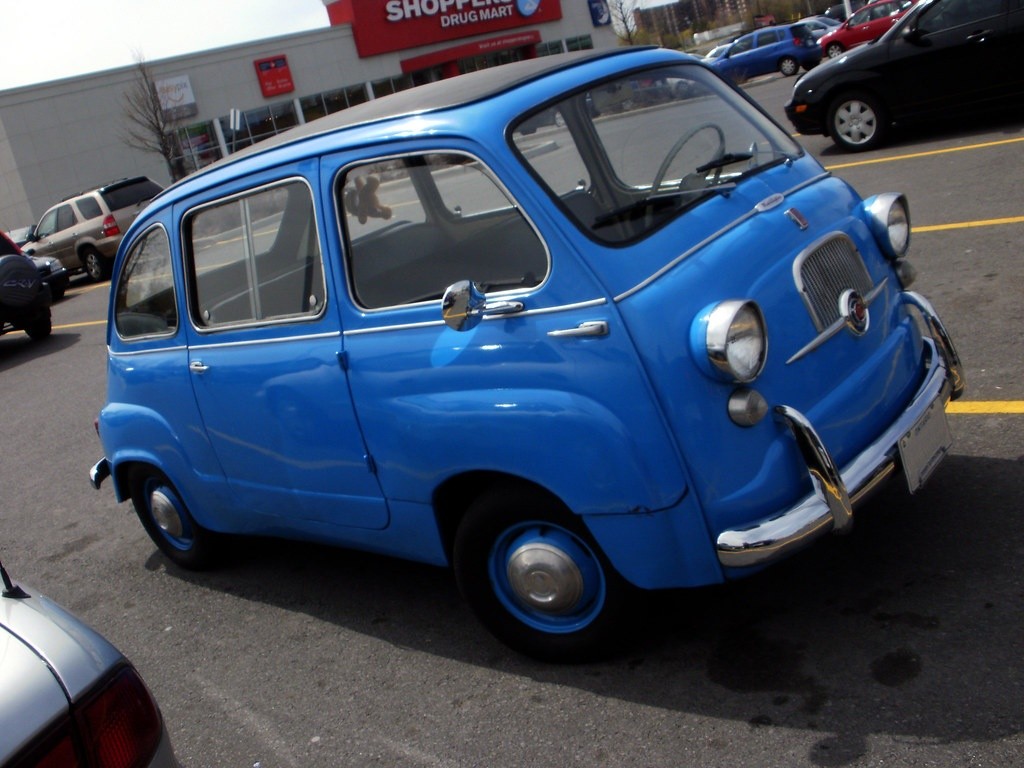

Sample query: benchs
[199,220,413,326]
[355,191,625,306]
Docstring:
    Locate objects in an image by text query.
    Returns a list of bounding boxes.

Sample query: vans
[87,44,970,664]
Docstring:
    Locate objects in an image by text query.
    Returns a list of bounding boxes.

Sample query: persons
[740,31,745,36]
[768,20,776,26]
[670,80,692,102]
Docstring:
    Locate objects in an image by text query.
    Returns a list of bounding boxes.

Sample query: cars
[4,224,38,248]
[0,572,183,768]
[512,0,900,138]
[24,248,70,301]
[782,0,1024,154]
[815,0,922,58]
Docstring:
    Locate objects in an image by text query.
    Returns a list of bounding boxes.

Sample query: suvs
[19,175,168,284]
[0,228,53,340]
